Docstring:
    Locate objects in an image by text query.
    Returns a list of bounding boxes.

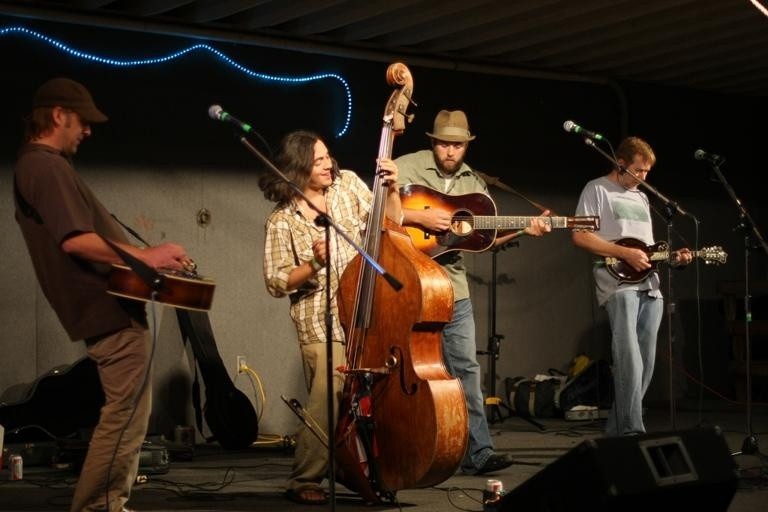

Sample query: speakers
[483,425,741,512]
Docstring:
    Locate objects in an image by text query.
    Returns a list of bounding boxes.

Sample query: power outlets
[237,355,247,376]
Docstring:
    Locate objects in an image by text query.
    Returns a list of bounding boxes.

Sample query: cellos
[334,63,468,508]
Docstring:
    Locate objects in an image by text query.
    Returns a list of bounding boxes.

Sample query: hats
[34,78,108,123]
[426,110,476,142]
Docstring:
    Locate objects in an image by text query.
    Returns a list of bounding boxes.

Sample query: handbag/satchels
[506,376,567,419]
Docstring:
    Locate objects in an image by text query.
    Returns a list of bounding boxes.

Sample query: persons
[13,77,191,511]
[570,135,693,436]
[263,124,404,505]
[393,109,552,476]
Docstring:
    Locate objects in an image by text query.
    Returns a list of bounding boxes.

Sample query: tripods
[477,241,547,431]
[712,169,768,462]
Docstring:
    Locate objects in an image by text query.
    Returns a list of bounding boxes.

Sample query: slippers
[287,485,326,504]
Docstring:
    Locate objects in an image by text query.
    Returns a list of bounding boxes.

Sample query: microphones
[694,149,720,168]
[208,104,257,135]
[563,120,609,144]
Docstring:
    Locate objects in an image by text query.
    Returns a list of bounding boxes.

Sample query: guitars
[605,238,730,284]
[398,185,600,259]
[107,258,216,312]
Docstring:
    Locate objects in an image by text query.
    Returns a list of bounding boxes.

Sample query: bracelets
[308,255,322,271]
[517,226,525,236]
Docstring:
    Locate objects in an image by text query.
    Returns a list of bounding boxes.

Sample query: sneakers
[476,453,514,475]
